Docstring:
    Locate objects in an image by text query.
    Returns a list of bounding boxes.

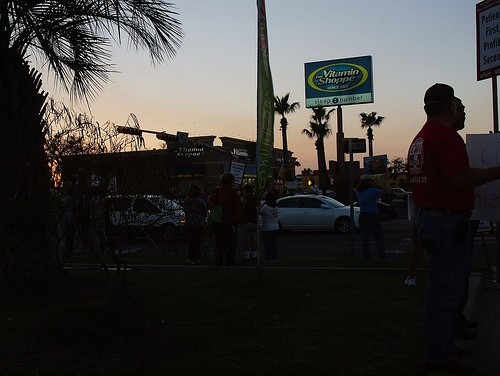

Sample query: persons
[452,96,479,339]
[310,180,336,198]
[183,174,258,271]
[59,176,106,256]
[408,83,500,376]
[356,174,389,262]
[261,186,279,260]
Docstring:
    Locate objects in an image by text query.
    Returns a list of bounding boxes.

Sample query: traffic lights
[307,179,315,185]
[117,126,141,135]
[157,133,179,142]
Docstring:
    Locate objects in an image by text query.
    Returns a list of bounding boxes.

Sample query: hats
[425,84,461,104]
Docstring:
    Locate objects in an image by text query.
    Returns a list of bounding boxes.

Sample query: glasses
[456,106,464,112]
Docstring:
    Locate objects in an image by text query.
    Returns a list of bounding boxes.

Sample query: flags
[257,0,275,200]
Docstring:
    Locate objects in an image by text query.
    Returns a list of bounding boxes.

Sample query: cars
[355,199,398,222]
[101,193,210,242]
[390,188,412,200]
[257,193,362,235]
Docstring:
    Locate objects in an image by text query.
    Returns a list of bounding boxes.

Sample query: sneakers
[404,275,417,286]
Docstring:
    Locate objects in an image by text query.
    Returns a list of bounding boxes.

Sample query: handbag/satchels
[359,208,368,227]
[277,219,283,233]
[208,203,223,232]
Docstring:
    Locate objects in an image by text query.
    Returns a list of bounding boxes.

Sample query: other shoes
[186,259,202,264]
[363,257,372,262]
[454,343,473,356]
[421,355,475,376]
[454,319,479,339]
[264,256,272,260]
[241,257,257,264]
[380,258,392,264]
